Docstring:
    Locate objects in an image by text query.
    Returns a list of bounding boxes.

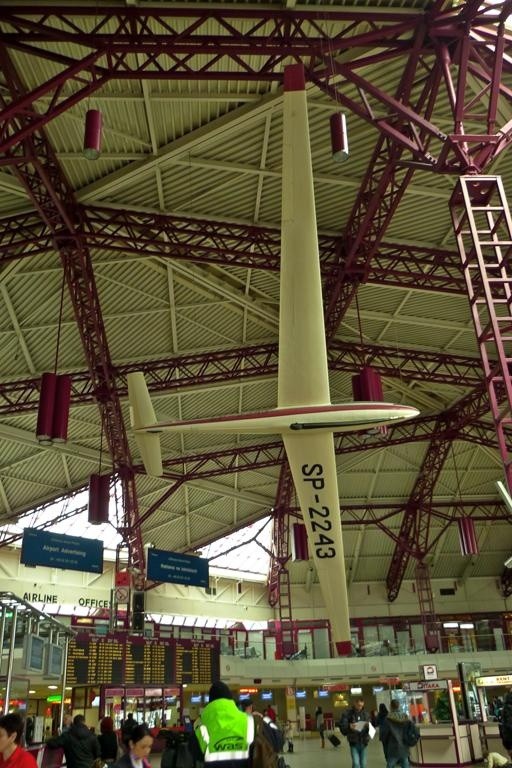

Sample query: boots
[321,739,325,749]
[287,743,293,752]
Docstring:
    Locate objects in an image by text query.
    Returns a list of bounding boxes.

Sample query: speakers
[133,592,145,611]
[133,613,144,633]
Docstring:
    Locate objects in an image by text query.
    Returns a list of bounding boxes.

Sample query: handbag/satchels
[262,716,285,752]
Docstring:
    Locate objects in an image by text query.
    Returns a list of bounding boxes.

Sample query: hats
[101,717,113,733]
[209,681,231,702]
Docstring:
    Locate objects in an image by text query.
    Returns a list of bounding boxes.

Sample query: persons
[378,698,412,768]
[0,696,289,767]
[486,692,506,722]
[500,687,512,759]
[339,696,375,768]
[376,703,390,762]
[313,704,326,748]
[185,679,257,768]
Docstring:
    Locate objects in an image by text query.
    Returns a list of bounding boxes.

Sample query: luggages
[328,735,340,747]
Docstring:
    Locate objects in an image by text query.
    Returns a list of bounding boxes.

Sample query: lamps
[350,289,387,440]
[84,15,103,163]
[35,255,70,446]
[329,18,351,163]
[449,442,479,557]
[290,488,309,562]
[89,406,110,526]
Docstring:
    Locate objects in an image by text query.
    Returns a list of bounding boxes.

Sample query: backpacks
[338,714,349,737]
[403,721,420,747]
[253,715,278,768]
